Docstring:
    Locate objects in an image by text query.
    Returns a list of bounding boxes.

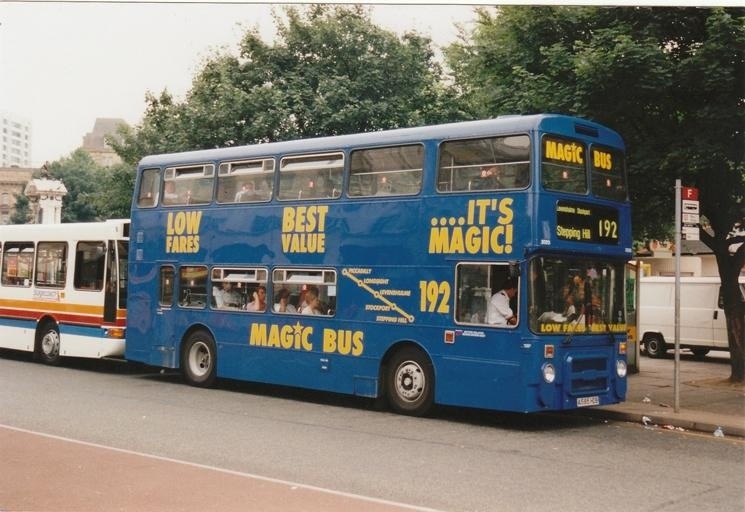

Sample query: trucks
[640,277,745,359]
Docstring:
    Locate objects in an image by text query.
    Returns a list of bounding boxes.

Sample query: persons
[234,182,259,202]
[321,179,335,198]
[487,282,518,326]
[154,181,179,207]
[216,280,331,316]
[477,167,506,190]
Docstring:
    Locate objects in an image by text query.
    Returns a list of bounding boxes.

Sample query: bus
[0,220,129,366]
[125,111,634,418]
